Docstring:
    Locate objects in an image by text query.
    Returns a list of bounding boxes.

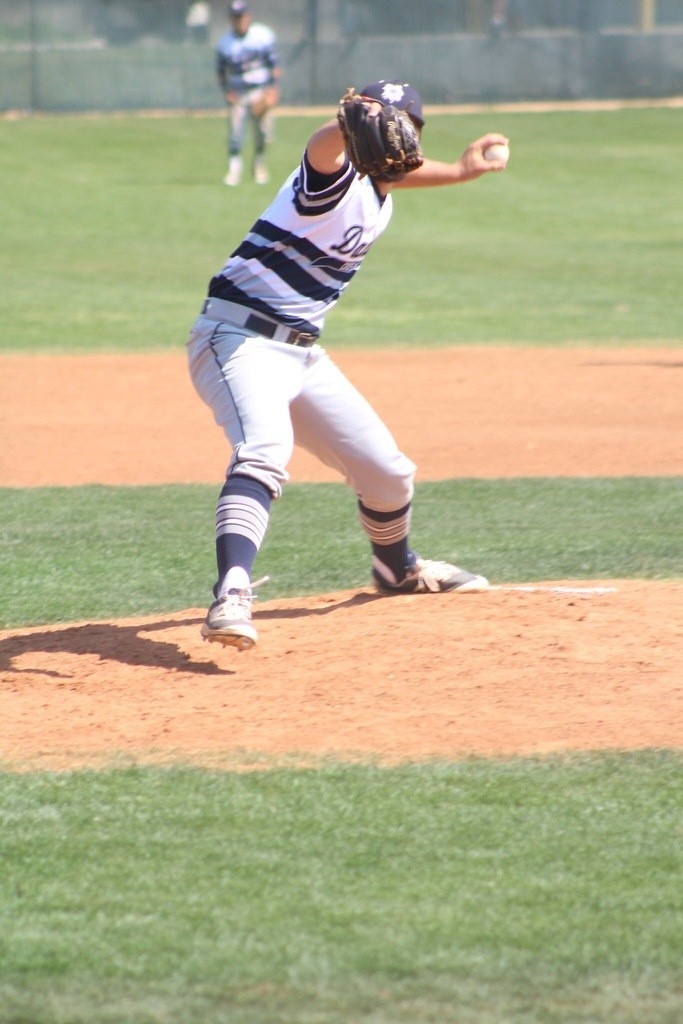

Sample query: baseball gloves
[338,86,423,181]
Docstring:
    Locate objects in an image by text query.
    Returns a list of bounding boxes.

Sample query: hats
[228,0,249,15]
[361,79,425,128]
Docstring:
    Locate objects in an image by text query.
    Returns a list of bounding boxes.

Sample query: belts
[244,314,318,347]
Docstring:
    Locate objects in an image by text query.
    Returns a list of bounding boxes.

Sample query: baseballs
[484,144,510,165]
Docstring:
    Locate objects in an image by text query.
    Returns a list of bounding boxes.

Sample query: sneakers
[372,558,492,594]
[201,592,259,653]
[222,170,241,186]
[255,168,269,185]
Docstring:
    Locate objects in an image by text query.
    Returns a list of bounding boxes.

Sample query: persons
[212,1,285,188]
[180,76,513,650]
